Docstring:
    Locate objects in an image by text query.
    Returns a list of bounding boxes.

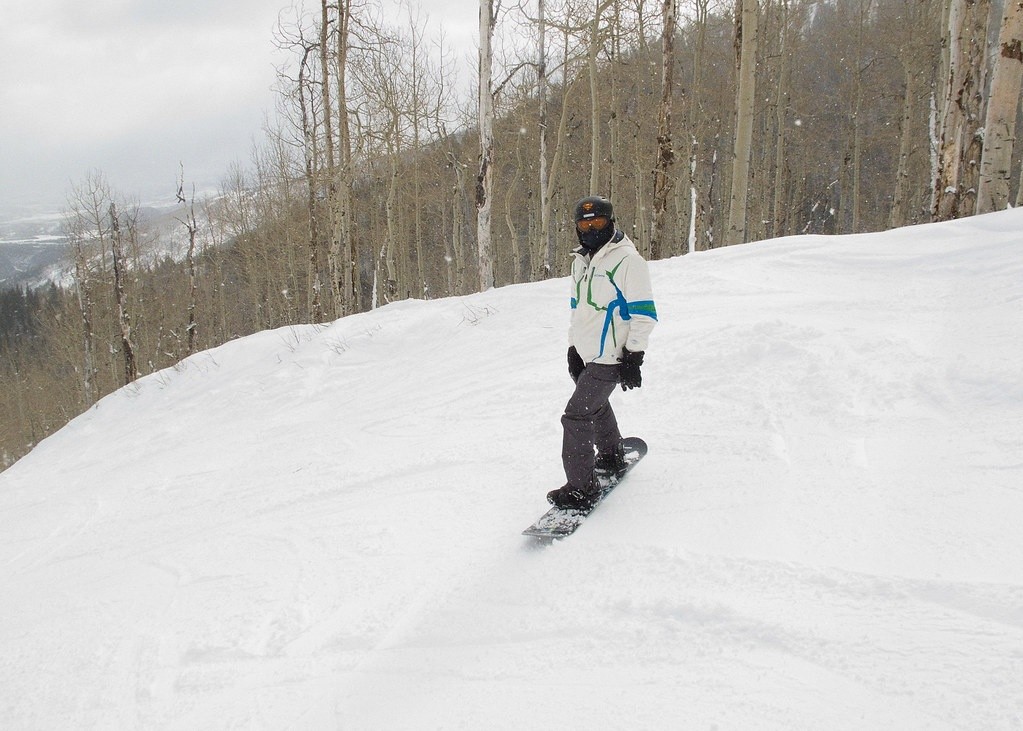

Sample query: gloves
[616,346,645,392]
[567,346,585,384]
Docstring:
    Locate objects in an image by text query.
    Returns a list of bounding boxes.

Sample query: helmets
[575,196,616,252]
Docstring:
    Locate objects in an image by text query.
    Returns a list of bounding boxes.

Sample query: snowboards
[521,436,648,539]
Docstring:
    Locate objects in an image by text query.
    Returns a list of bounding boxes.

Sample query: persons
[547,196,658,508]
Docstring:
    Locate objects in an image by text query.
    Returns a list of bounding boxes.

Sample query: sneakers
[547,480,602,510]
[594,443,625,473]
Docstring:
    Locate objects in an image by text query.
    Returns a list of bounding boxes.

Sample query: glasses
[577,217,609,232]
